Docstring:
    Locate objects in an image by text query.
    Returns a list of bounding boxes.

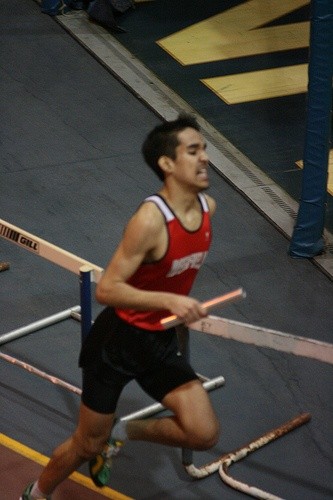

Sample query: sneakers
[90,417,123,487]
[19,483,49,500]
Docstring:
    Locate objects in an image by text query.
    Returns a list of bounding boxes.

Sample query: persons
[18,112,218,500]
